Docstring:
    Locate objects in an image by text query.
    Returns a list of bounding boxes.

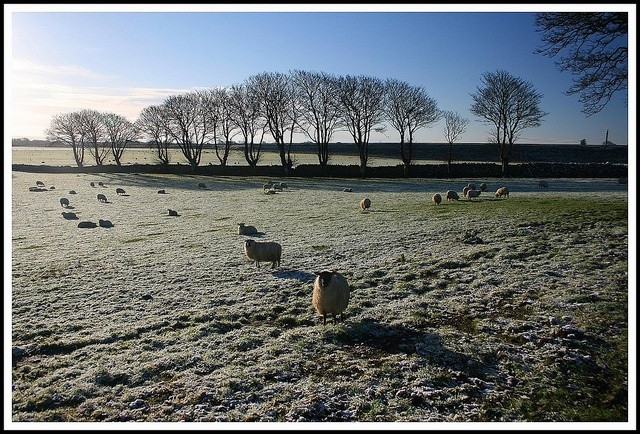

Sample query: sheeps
[359,198,372,211]
[167,209,181,216]
[61,212,79,220]
[431,181,511,206]
[27,178,128,207]
[78,219,114,230]
[243,238,282,268]
[312,266,352,324]
[238,222,257,235]
[263,181,288,195]
